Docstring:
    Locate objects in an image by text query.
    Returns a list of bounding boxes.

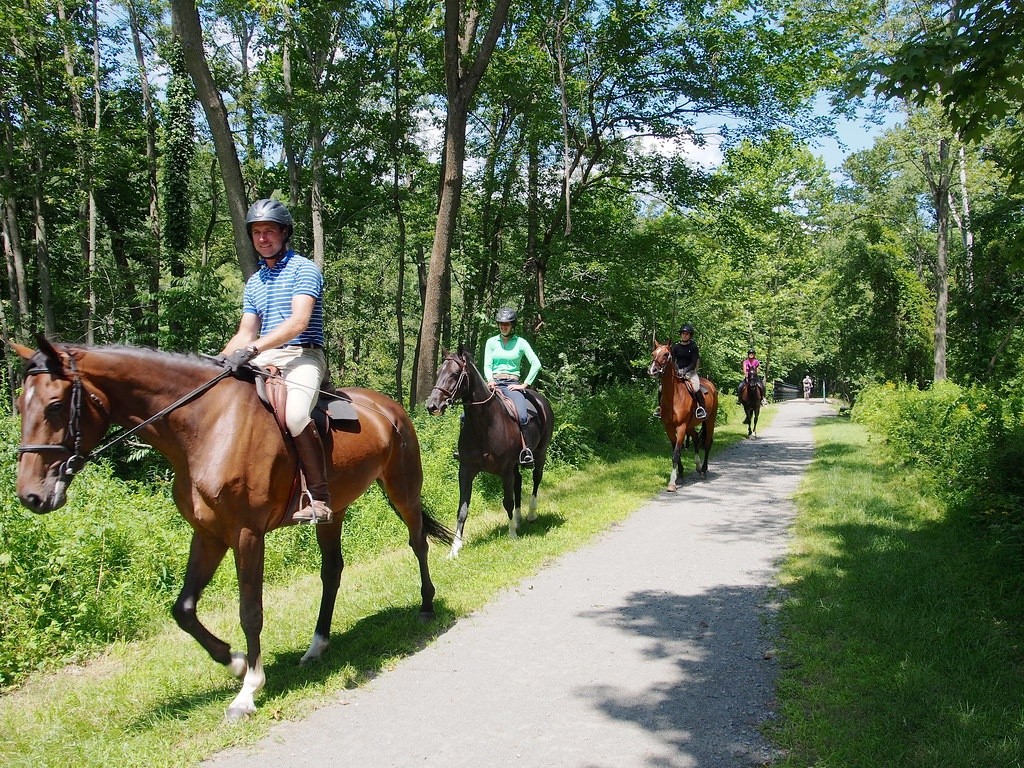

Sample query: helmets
[676,324,694,334]
[245,198,293,241]
[495,308,515,322]
[747,349,755,354]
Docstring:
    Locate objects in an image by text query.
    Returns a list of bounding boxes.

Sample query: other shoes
[697,409,706,417]
[736,401,741,405]
[523,451,534,469]
[761,398,767,405]
[453,453,460,461]
[654,412,660,416]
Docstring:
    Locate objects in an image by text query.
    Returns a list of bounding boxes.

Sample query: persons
[484,306,542,469]
[736,349,768,405]
[652,323,708,418]
[213,198,334,526]
[803,375,813,394]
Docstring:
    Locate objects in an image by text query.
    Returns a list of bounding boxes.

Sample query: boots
[292,419,332,525]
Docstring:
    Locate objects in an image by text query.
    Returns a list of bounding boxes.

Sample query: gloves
[223,346,258,375]
[678,368,688,376]
[209,353,226,367]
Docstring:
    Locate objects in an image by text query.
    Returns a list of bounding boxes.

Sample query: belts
[492,373,519,381]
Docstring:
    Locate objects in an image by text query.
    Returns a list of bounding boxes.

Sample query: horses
[739,363,762,440]
[0,331,466,723]
[647,334,719,493]
[425,337,556,561]
